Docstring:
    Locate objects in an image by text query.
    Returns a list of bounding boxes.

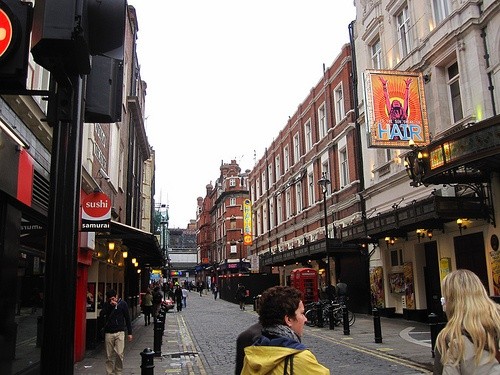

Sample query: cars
[139,292,172,306]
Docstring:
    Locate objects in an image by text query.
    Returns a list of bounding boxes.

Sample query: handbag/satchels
[101,328,105,337]
[245,290,249,297]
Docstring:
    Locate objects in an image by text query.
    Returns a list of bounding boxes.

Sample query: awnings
[111,220,166,267]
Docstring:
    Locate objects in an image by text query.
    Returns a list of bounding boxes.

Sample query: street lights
[317,171,331,298]
[199,241,204,263]
[237,237,243,271]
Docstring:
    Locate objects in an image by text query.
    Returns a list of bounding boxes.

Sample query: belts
[105,329,124,333]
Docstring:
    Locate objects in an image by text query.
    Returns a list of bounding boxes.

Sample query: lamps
[457,218,467,234]
[122,252,128,259]
[109,242,115,250]
[384,237,395,249]
[427,229,433,240]
[403,138,424,187]
[416,230,425,242]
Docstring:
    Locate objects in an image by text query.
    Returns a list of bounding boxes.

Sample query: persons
[142,278,188,326]
[16,268,44,314]
[99,289,132,375]
[184,279,209,296]
[237,284,247,311]
[434,269,500,375]
[235,286,330,375]
[213,284,218,300]
[322,279,349,311]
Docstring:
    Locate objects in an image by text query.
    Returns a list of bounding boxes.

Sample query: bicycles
[305,295,355,327]
